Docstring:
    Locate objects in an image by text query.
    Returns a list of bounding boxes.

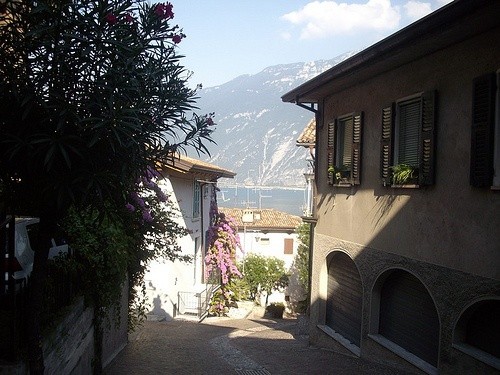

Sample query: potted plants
[328,162,352,181]
[388,162,419,185]
